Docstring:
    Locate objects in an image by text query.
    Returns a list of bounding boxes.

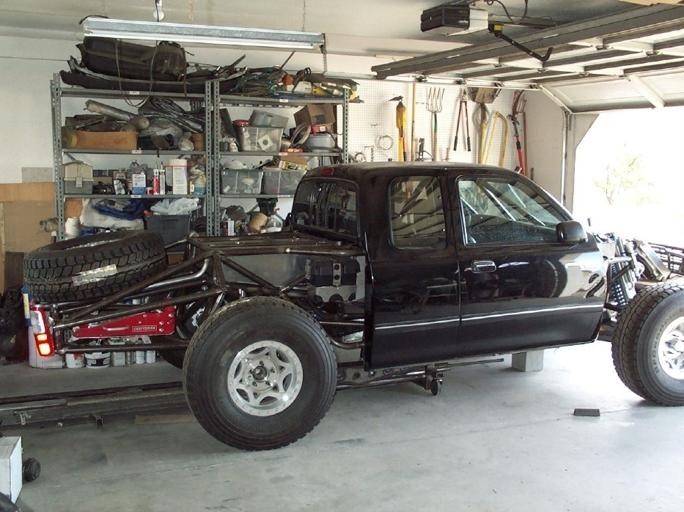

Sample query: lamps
[153,0,165,21]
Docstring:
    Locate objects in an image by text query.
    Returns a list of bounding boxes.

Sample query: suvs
[19,160,684,452]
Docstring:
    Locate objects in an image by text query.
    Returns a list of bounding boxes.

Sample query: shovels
[474,88,498,165]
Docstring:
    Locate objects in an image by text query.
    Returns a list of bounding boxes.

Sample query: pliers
[453,90,471,151]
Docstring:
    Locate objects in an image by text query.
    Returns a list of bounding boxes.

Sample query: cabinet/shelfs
[213,78,349,237]
[50,72,212,241]
